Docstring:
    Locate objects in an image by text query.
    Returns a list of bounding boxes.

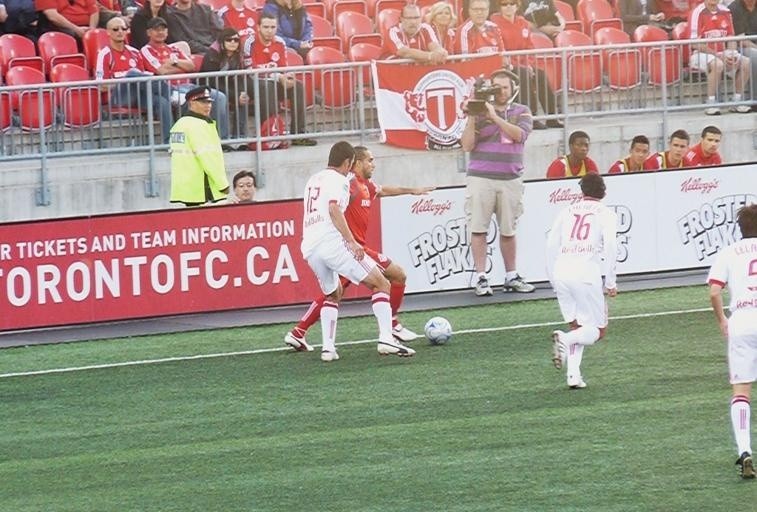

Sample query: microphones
[506,91,520,104]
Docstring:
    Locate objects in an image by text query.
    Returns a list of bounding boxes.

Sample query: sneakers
[284,333,314,353]
[551,328,565,371]
[566,369,588,388]
[728,104,751,115]
[320,350,340,362]
[390,323,418,341]
[735,452,757,481]
[474,276,494,297]
[290,136,317,146]
[704,107,722,117]
[503,274,536,294]
[377,337,416,358]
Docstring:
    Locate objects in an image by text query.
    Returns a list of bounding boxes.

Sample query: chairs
[337,10,375,48]
[83,27,128,64]
[532,32,559,91]
[673,19,715,73]
[0,32,45,77]
[278,51,316,112]
[577,0,624,40]
[552,0,582,32]
[37,31,85,71]
[379,10,410,41]
[6,67,59,130]
[305,45,356,107]
[636,23,681,88]
[352,42,385,91]
[50,64,104,125]
[555,30,599,96]
[308,15,335,38]
[598,28,639,88]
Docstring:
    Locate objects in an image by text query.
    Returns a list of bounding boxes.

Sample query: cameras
[466,77,503,114]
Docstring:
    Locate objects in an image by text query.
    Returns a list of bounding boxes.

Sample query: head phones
[491,68,520,94]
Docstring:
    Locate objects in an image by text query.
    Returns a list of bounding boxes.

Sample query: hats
[186,88,215,102]
[144,17,167,30]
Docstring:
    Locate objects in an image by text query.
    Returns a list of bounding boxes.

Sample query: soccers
[424,317,452,344]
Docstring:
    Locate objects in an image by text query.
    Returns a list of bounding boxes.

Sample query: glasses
[500,2,516,7]
[224,37,240,43]
[472,7,487,12]
[111,26,129,31]
[402,16,420,20]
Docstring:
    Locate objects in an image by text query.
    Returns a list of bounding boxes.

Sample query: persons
[547,131,599,179]
[95,17,186,145]
[690,126,722,165]
[490,0,566,130]
[243,13,317,146]
[546,170,618,388]
[34,0,99,51]
[459,72,535,296]
[300,141,416,361]
[262,0,314,58]
[618,0,688,36]
[727,0,757,111]
[707,204,757,479]
[378,3,448,66]
[198,28,249,139]
[130,0,175,50]
[284,146,418,352]
[424,1,456,55]
[608,136,653,174]
[217,0,261,37]
[168,86,240,206]
[140,17,231,137]
[233,171,257,203]
[688,0,752,115]
[453,0,510,66]
[0,0,41,43]
[158,0,224,53]
[646,130,704,170]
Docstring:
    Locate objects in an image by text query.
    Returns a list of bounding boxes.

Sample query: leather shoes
[546,118,565,128]
[532,119,548,129]
